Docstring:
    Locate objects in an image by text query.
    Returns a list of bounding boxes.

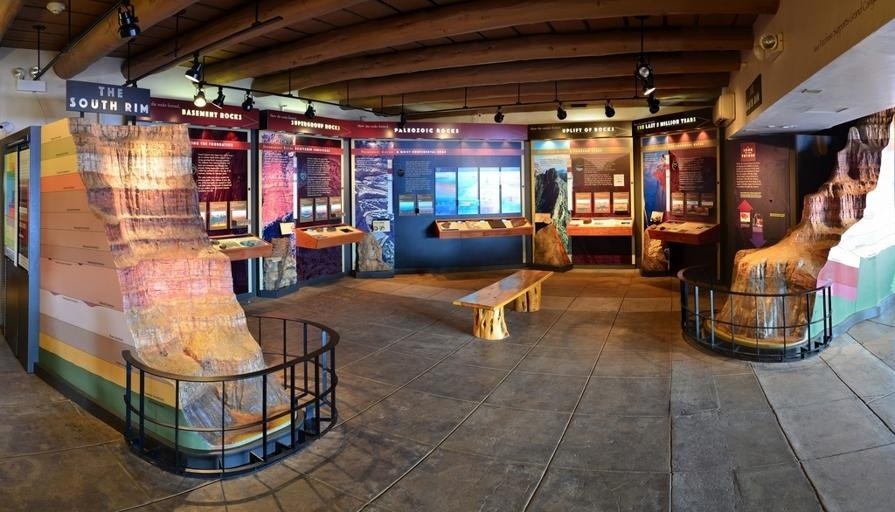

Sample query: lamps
[398,93,408,126]
[241,91,255,112]
[604,100,616,117]
[192,84,207,108]
[116,3,140,39]
[185,57,202,83]
[642,82,655,96]
[753,33,784,64]
[495,106,505,123]
[647,96,660,114]
[557,102,567,120]
[634,16,652,80]
[304,100,316,120]
[211,86,225,109]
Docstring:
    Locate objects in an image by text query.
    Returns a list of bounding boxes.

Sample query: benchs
[452,270,554,341]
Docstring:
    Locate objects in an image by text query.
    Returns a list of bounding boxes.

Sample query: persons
[535,168,560,232]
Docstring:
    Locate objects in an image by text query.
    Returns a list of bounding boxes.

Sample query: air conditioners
[713,93,735,128]
